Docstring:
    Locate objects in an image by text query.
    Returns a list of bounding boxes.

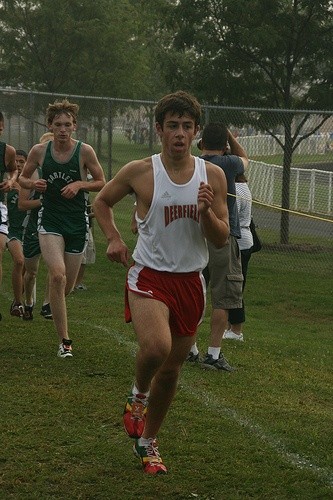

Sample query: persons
[17,101,106,358]
[93,90,230,476]
[0,111,96,322]
[123,113,151,144]
[187,121,253,371]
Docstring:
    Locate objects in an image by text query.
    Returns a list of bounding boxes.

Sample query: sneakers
[11,297,23,317]
[40,303,53,320]
[23,300,34,321]
[132,439,167,477]
[56,338,72,358]
[203,352,238,372]
[123,381,150,439]
[184,352,199,365]
[222,329,243,340]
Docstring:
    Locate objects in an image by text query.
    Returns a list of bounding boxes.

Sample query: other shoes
[74,283,86,290]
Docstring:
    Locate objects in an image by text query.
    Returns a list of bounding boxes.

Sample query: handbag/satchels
[249,218,262,253]
